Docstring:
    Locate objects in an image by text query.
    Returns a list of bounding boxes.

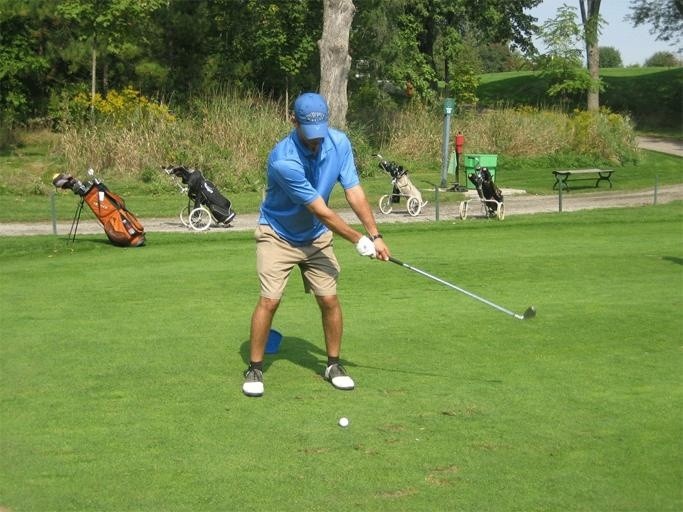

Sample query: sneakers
[324,362,354,390]
[242,366,264,397]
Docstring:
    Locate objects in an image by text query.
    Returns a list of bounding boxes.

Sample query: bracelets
[370,234,383,241]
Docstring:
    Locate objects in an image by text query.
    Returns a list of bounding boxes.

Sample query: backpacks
[391,168,423,209]
[476,169,502,211]
[182,170,236,226]
[83,182,147,247]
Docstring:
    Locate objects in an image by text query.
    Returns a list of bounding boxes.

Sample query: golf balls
[340,418,348,426]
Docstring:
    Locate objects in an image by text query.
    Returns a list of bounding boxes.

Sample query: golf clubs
[389,257,536,320]
[52,173,88,194]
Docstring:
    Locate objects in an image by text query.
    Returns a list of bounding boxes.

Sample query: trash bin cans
[463,154,499,190]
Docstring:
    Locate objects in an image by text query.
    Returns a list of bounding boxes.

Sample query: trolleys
[371,153,428,216]
[160,162,236,232]
[458,156,506,222]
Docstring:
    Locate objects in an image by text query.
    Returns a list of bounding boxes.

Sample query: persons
[242,93,390,396]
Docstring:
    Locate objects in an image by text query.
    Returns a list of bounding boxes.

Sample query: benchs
[552,169,615,192]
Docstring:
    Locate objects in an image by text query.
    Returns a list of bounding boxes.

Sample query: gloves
[355,235,376,257]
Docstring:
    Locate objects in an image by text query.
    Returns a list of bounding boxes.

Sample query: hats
[294,92,330,139]
